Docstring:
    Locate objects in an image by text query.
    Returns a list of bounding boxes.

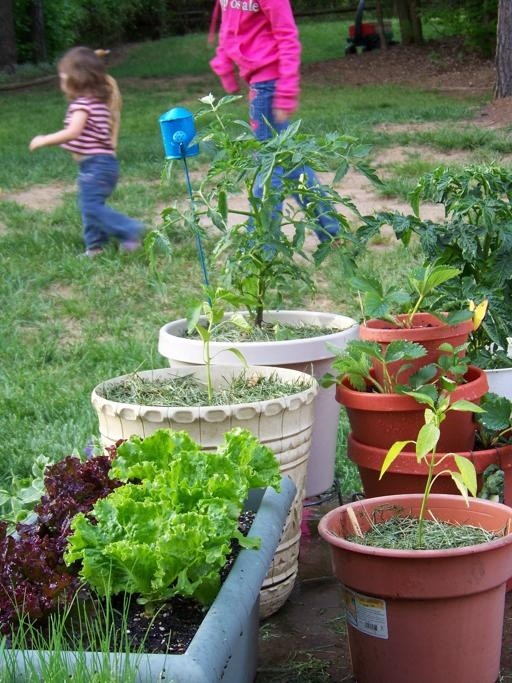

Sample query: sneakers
[73,240,141,262]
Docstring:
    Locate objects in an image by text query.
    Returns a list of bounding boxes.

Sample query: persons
[208,0,351,262]
[28,46,149,258]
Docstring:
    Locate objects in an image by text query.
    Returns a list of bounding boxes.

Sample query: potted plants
[90,92,386,502]
[320,160,511,683]
[92,283,319,619]
[2,425,298,683]
